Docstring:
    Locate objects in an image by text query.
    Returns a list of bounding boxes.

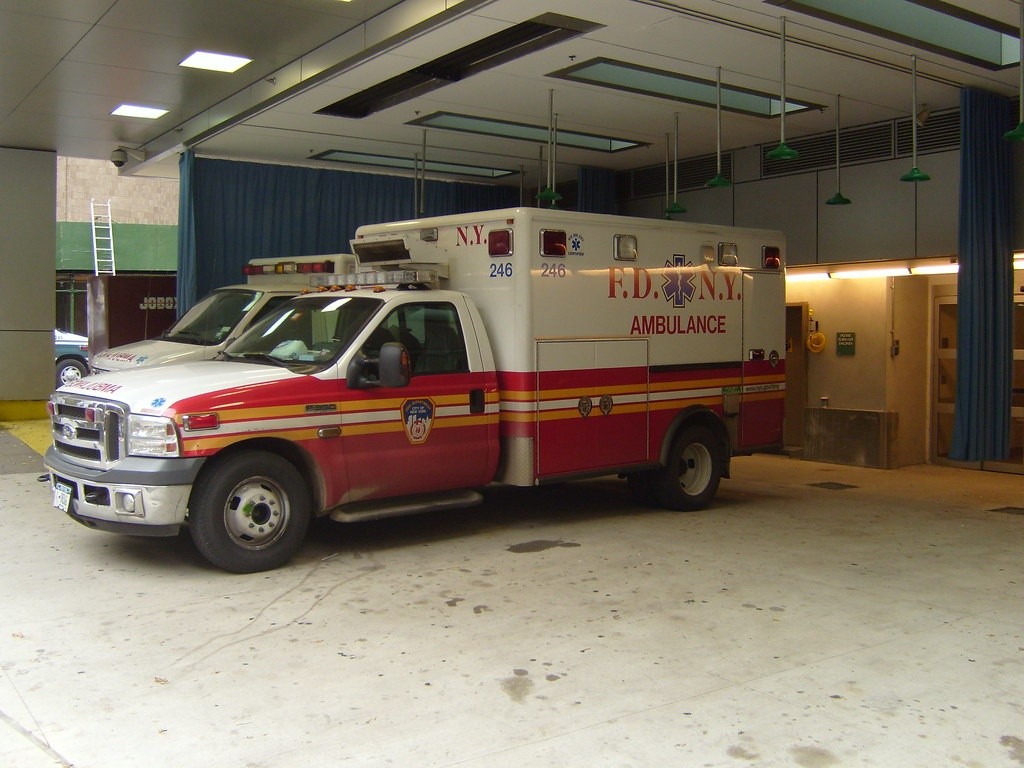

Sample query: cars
[54,329,90,391]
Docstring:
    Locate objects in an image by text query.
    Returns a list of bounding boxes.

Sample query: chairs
[412,324,465,372]
[345,323,396,382]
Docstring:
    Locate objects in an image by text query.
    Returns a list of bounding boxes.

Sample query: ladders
[91,196,116,277]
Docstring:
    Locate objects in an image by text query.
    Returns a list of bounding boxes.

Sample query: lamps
[706,67,732,188]
[824,95,852,205]
[900,54,932,182]
[916,104,930,126]
[765,16,798,160]
[1002,0,1024,141]
[534,88,562,210]
[660,111,687,221]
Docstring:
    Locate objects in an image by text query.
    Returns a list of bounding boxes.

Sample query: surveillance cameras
[112,150,128,168]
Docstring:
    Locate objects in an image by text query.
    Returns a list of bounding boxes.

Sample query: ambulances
[85,254,425,381]
[37,205,790,574]
